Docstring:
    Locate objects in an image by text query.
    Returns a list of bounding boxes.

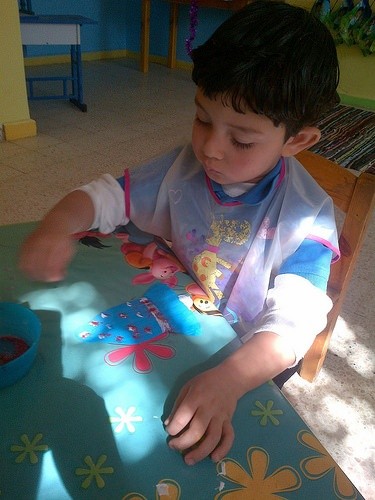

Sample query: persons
[20,1,344,465]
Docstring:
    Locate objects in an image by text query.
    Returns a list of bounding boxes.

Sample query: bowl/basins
[0,303,42,387]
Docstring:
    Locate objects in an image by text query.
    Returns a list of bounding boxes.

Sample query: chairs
[293,150,375,382]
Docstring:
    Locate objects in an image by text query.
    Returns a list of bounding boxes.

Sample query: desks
[0,220,366,500]
[140,1,255,74]
[21,14,97,113]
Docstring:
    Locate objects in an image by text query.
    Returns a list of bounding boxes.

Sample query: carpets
[306,105,375,177]
